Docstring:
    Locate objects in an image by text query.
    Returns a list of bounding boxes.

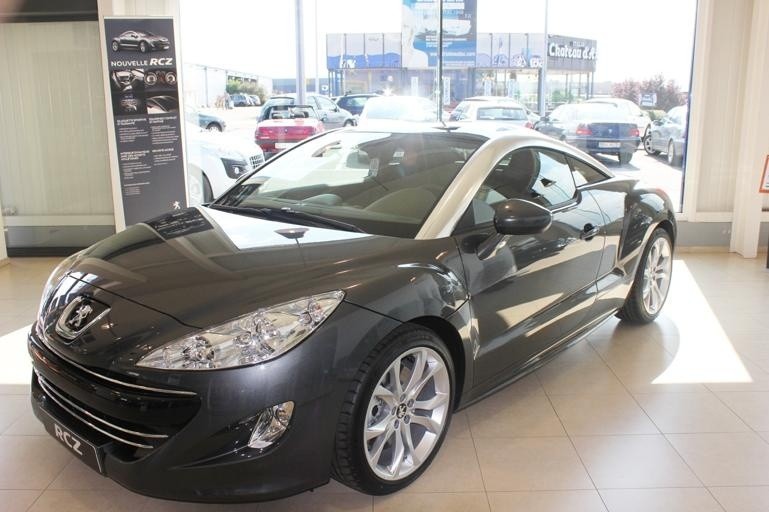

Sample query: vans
[361,94,450,125]
[230,93,261,106]
[256,92,356,132]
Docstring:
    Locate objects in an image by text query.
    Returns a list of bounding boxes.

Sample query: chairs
[494,149,552,208]
[294,111,305,118]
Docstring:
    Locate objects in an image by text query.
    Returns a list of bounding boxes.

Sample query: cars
[332,93,379,113]
[254,104,329,156]
[26,121,675,505]
[449,95,538,129]
[532,97,691,163]
[189,121,266,207]
